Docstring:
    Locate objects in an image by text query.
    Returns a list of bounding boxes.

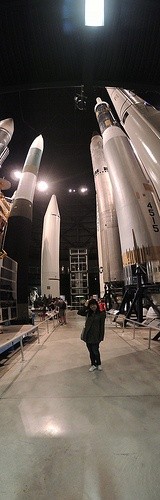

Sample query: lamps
[73,84,90,112]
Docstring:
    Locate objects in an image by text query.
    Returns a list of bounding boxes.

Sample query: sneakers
[89,366,96,372]
[98,365,102,370]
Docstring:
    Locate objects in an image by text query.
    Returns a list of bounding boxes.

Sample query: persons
[55,298,67,325]
[77,299,106,372]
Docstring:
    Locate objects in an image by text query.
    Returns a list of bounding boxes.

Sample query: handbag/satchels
[80,328,86,342]
